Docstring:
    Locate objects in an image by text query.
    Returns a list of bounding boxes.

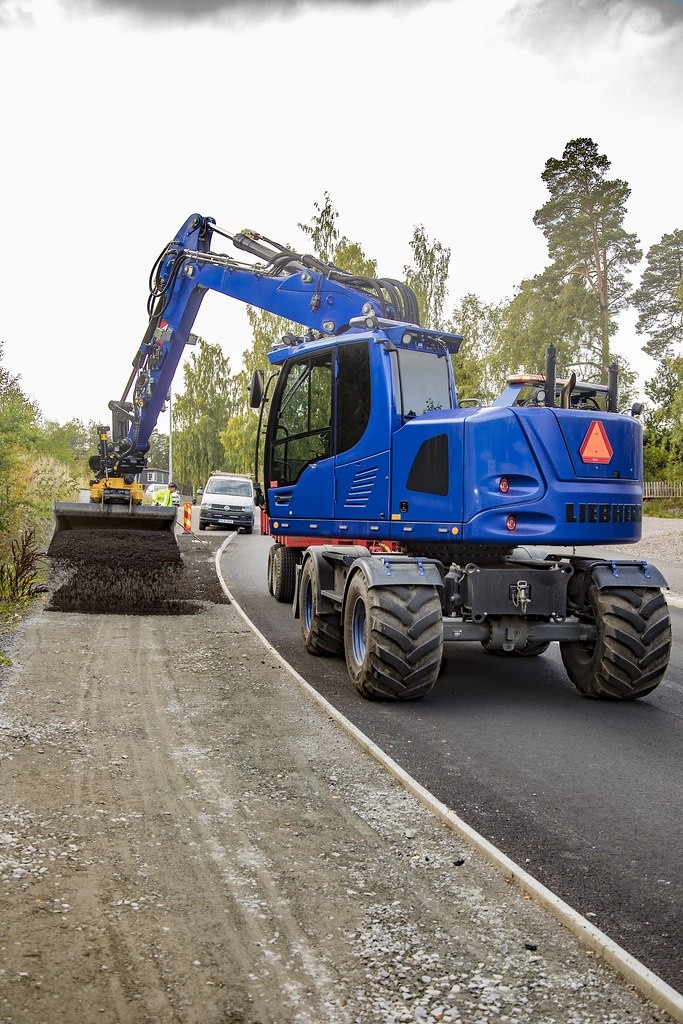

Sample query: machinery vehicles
[46,214,674,704]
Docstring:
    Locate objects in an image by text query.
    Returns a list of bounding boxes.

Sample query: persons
[152,482,178,507]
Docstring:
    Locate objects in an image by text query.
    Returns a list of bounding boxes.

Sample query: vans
[197,475,255,534]
